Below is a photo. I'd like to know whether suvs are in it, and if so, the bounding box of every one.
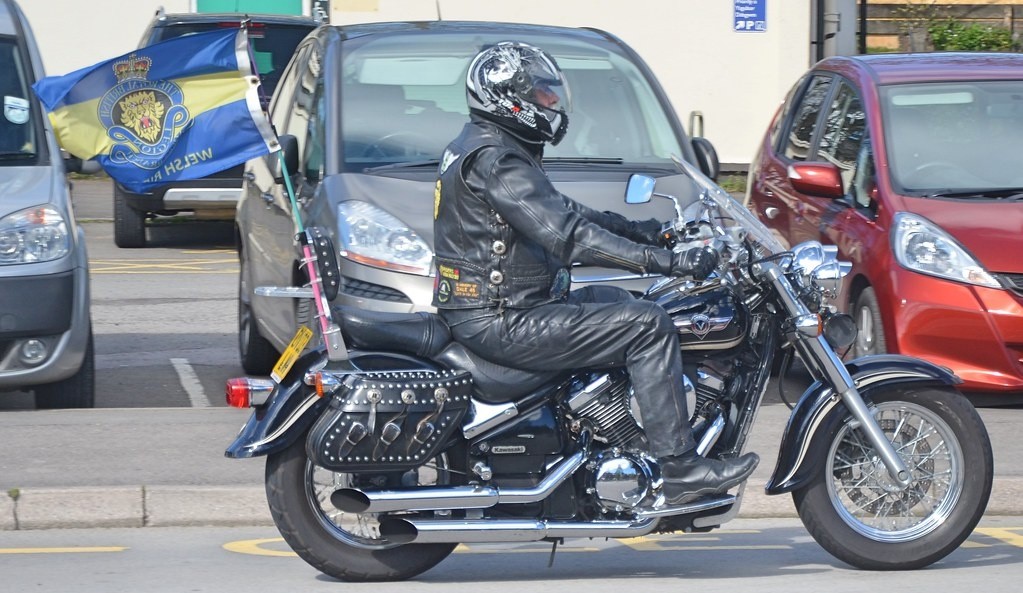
[111,8,330,248]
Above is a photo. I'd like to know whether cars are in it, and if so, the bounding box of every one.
[233,20,802,407]
[743,51,1021,413]
[0,1,97,410]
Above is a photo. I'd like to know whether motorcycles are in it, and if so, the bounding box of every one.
[222,132,993,582]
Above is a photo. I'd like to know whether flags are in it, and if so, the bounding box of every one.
[31,28,282,197]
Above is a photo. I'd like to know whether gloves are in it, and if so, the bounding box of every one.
[671,246,720,284]
[627,217,668,250]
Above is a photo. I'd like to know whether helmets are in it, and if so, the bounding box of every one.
[465,40,572,147]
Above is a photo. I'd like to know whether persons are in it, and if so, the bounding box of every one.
[432,40,760,508]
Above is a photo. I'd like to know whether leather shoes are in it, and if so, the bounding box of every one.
[654,449,762,506]
